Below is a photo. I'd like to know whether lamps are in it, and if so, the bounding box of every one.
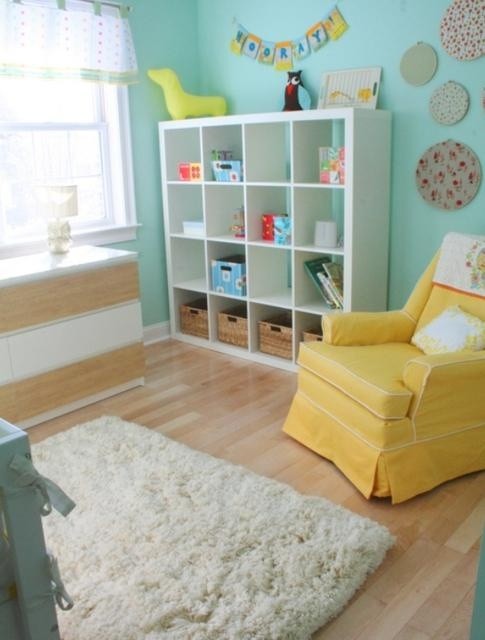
[33,183,80,254]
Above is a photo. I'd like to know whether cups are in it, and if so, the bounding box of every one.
[314,220,337,249]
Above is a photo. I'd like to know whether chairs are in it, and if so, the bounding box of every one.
[282,232,485,503]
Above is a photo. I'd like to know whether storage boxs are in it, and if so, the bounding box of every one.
[210,158,243,182]
[179,298,323,360]
[211,252,246,297]
[317,145,345,184]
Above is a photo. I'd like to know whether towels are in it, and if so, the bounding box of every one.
[432,231,485,298]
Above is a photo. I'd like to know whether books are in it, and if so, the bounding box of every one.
[303,257,343,311]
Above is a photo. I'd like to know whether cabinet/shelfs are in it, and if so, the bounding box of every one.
[156,106,392,373]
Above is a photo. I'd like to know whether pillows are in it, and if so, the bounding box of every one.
[409,303,485,353]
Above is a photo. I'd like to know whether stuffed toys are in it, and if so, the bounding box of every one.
[146,68,228,121]
[280,69,313,112]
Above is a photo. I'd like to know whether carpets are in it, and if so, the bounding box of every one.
[23,414,404,640]
